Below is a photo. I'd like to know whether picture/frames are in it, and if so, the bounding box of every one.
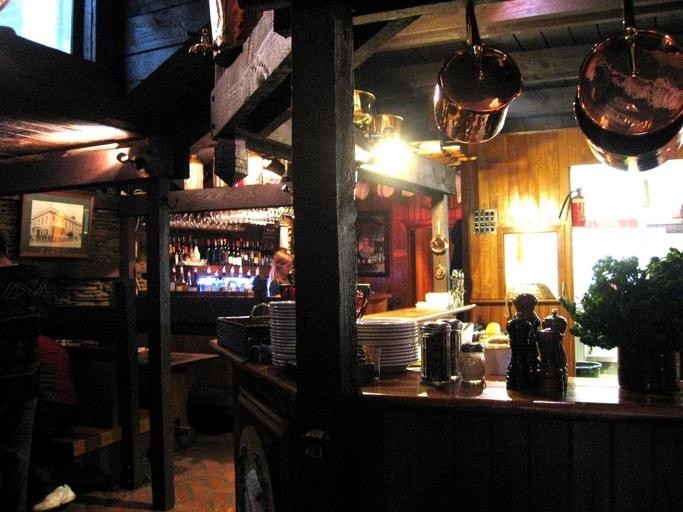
[355,210,389,277]
[18,192,95,260]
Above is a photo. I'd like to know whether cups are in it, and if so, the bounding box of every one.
[169,207,292,231]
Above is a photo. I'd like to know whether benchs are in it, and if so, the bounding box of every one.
[137,332,232,408]
[65,345,150,483]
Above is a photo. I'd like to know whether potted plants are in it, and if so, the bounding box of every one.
[559,248,683,393]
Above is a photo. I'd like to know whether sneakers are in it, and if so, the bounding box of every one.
[32,483,76,512]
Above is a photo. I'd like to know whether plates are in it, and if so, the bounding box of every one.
[270,300,297,369]
[355,317,419,376]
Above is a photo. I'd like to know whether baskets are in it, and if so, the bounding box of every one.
[216,303,270,358]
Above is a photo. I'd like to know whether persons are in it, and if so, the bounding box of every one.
[33,335,77,510]
[0,233,49,511]
[266,249,295,300]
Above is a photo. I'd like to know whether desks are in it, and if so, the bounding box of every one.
[138,349,220,450]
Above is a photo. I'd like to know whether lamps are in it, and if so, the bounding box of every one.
[354,90,405,167]
[281,184,293,196]
[261,158,285,176]
[117,153,143,169]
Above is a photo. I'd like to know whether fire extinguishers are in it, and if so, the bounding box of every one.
[558,187,586,227]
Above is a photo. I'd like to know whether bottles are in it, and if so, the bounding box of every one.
[170,266,263,294]
[460,342,485,385]
[168,231,275,266]
[417,319,460,386]
